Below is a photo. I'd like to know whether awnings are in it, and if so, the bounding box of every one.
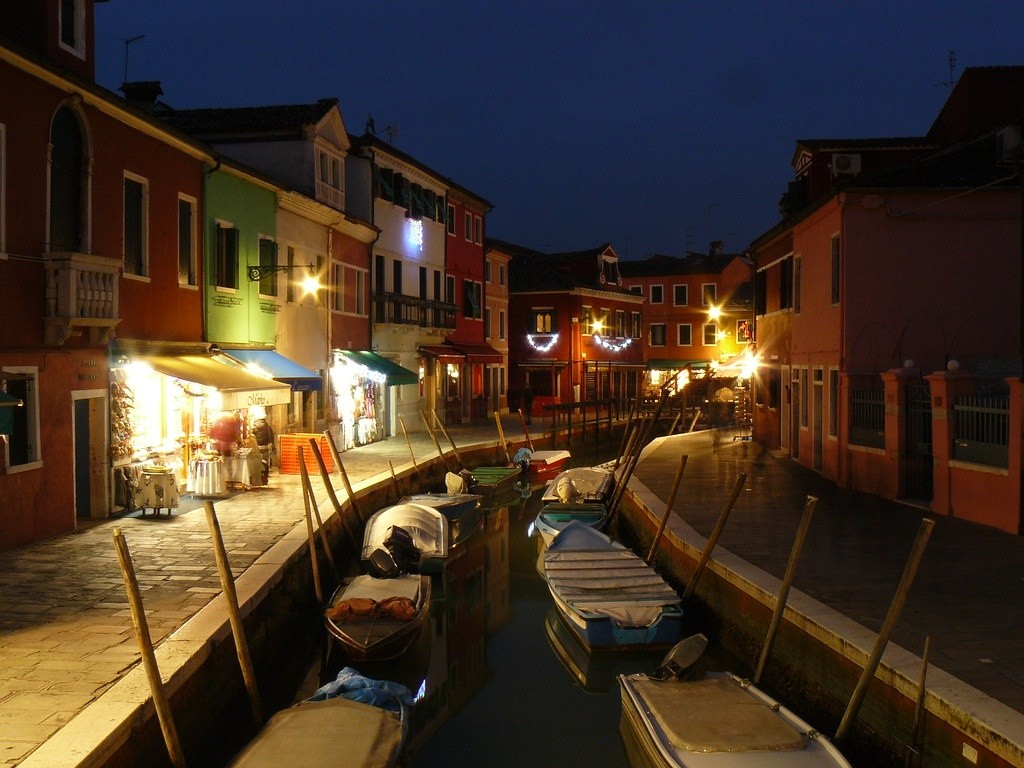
[518,361,568,367]
[136,351,322,410]
[585,361,647,372]
[334,349,419,386]
[645,359,714,370]
[420,345,504,364]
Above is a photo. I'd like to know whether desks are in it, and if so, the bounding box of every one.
[189,459,224,499]
[137,472,176,516]
[220,454,263,492]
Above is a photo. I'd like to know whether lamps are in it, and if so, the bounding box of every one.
[248,261,322,295]
[582,316,603,331]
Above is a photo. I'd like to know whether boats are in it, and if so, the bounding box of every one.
[614,633,852,768]
[534,501,608,547]
[510,449,570,476]
[463,464,523,497]
[322,547,434,666]
[544,518,686,659]
[229,666,412,768]
[541,466,614,507]
[402,493,485,520]
[360,500,449,574]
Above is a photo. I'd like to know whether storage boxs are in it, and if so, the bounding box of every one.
[277,432,335,475]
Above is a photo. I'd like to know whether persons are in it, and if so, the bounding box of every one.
[513,445,532,474]
[210,409,253,490]
[250,417,275,478]
[445,463,468,494]
